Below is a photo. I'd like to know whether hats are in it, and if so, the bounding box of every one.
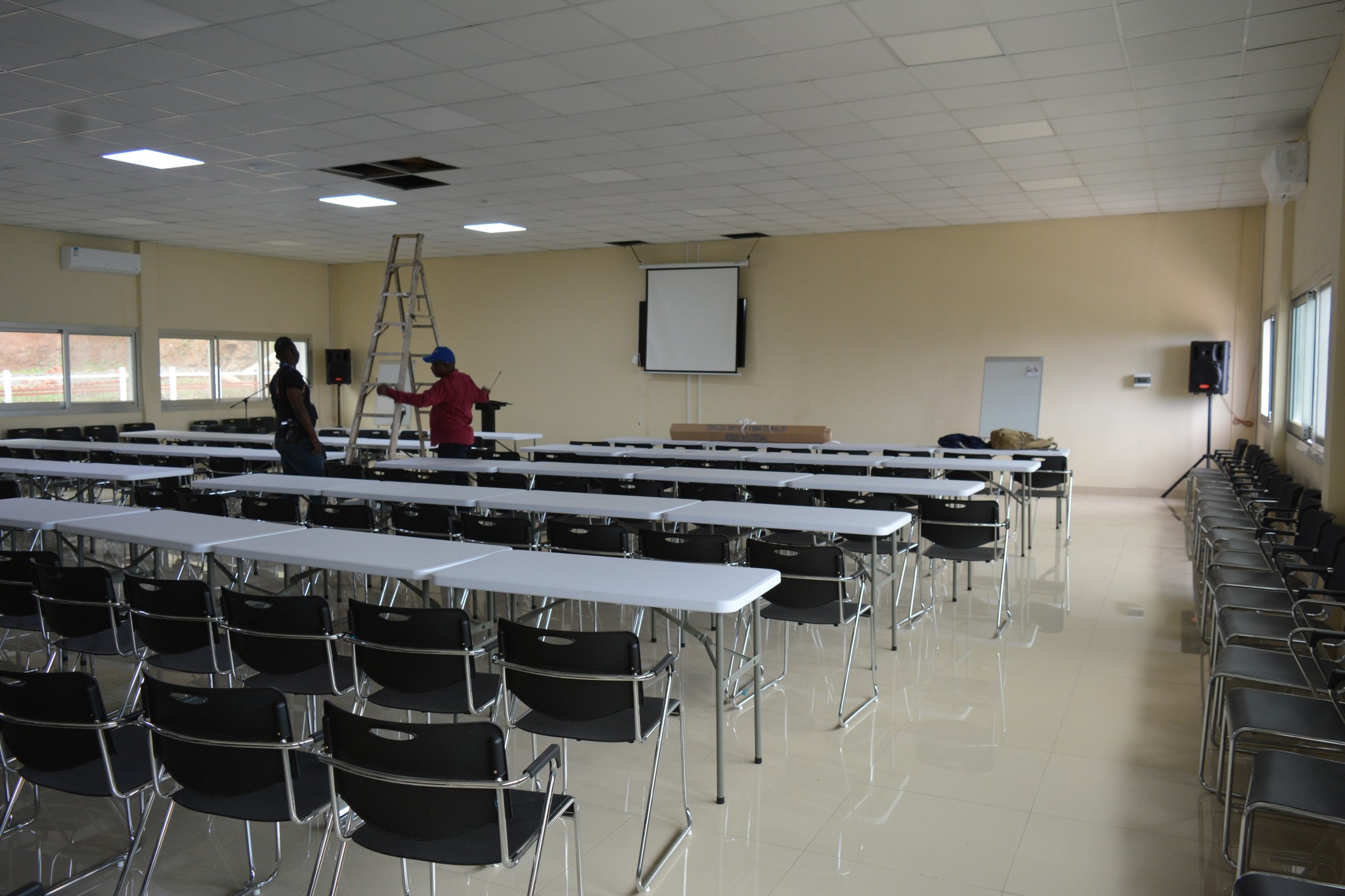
[422,346,456,363]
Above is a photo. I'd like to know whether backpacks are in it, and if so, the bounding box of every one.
[990,428,1058,450]
[937,433,993,449]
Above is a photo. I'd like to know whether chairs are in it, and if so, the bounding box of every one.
[0,414,1075,896]
[1186,437,1345,896]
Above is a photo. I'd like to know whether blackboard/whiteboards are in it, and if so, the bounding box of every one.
[977,356,1044,440]
[372,359,416,426]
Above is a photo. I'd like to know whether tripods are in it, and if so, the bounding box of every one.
[1158,394,1232,499]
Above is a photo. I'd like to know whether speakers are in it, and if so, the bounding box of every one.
[1188,340,1231,396]
[324,348,353,385]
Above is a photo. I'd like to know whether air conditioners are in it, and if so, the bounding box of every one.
[1260,140,1309,200]
[60,244,142,276]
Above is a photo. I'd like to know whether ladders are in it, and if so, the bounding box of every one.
[343,233,443,478]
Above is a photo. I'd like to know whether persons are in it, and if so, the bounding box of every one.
[376,346,491,486]
[268,335,327,526]
